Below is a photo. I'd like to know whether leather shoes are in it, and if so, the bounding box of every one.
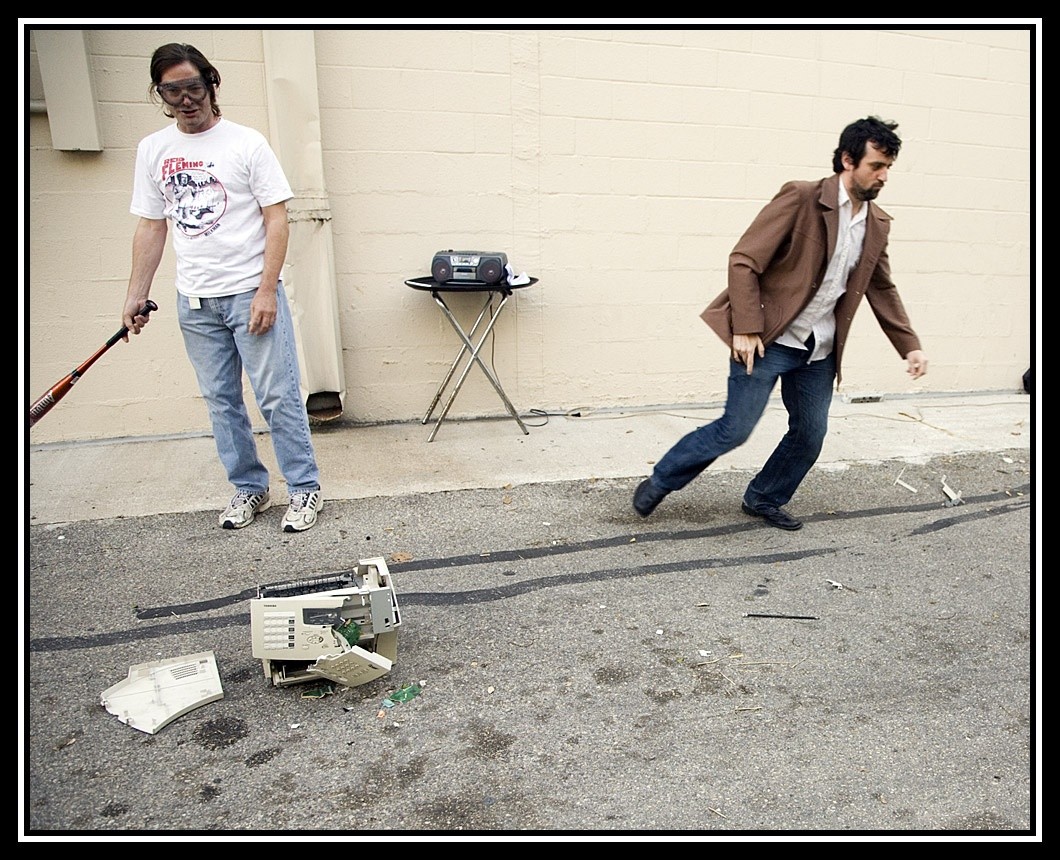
[632,478,665,516]
[741,502,803,530]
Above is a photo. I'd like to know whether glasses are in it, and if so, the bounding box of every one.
[156,79,208,106]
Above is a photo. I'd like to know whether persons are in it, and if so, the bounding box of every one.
[633,116,928,530]
[120,43,323,533]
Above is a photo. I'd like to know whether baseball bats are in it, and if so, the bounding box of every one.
[28,298,159,429]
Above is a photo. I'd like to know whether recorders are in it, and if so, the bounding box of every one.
[431,250,508,285]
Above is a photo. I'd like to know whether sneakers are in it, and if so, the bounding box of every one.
[281,490,323,531]
[218,490,271,529]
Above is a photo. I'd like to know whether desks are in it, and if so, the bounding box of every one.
[405,276,539,443]
